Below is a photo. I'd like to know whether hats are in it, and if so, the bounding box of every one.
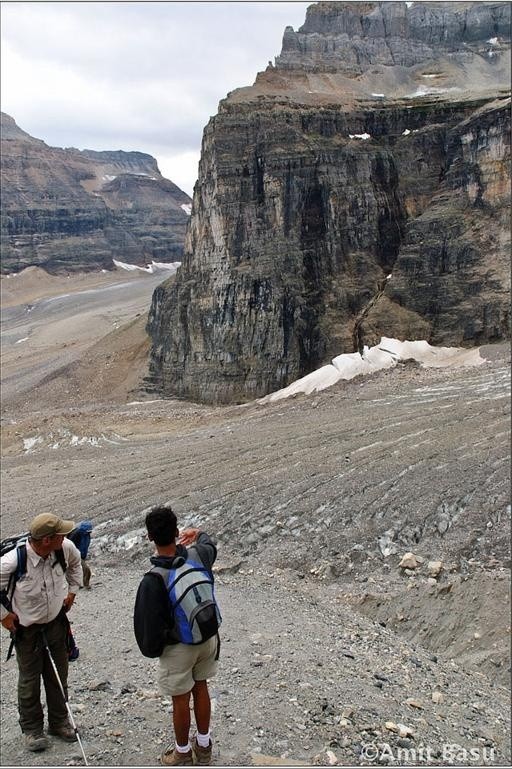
[29,512,75,541]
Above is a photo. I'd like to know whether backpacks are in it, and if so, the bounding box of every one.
[144,546,223,645]
[0,531,67,583]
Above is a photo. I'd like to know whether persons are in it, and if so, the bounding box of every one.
[133,503,222,765]
[1,511,86,752]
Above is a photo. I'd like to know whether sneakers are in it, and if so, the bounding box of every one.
[84,585,91,590]
[47,717,81,742]
[23,730,52,752]
[190,730,213,765]
[160,743,194,765]
